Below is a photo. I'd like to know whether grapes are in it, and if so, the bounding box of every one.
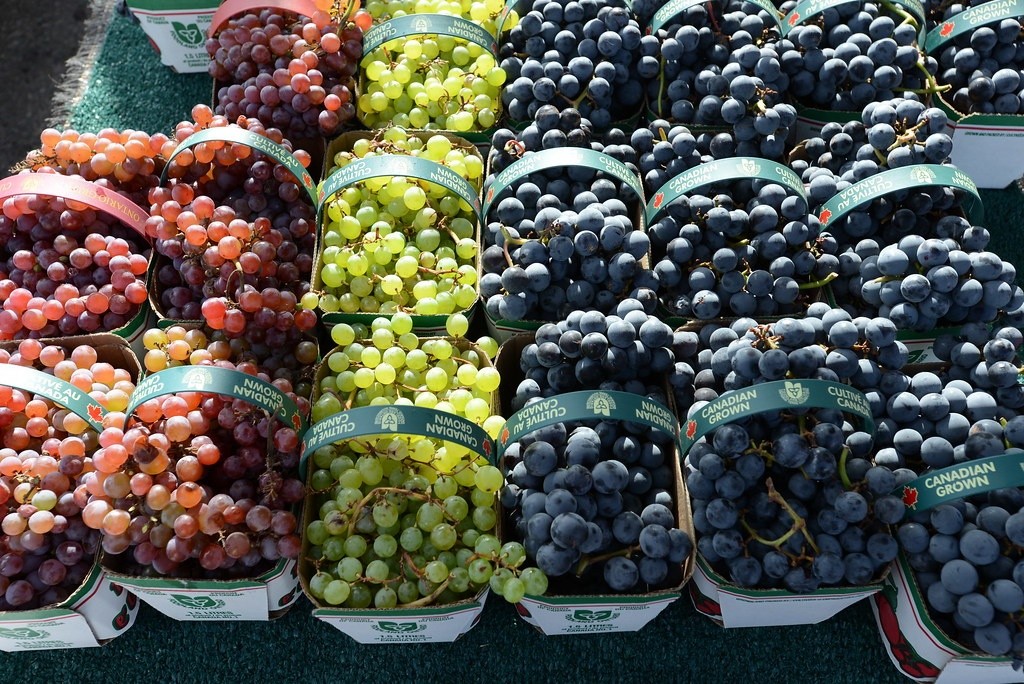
[0,0,1024,657]
